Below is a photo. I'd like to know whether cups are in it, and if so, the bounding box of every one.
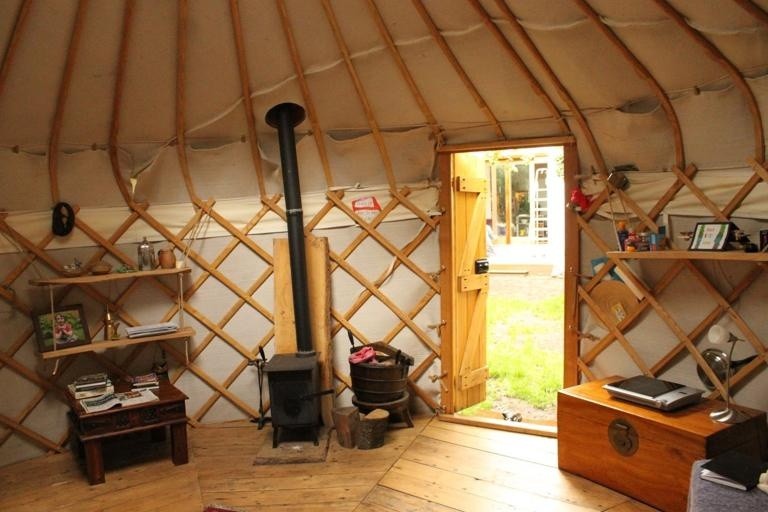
[152,362,168,383]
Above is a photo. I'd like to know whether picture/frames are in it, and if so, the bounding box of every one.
[31,303,91,353]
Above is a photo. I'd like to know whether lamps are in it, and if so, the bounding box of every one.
[704,320,749,423]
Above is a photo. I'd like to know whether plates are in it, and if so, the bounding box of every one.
[696,349,733,391]
[589,276,637,332]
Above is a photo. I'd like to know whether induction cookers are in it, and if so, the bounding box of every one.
[600,373,706,412]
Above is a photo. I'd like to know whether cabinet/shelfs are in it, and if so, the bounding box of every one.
[27,266,196,361]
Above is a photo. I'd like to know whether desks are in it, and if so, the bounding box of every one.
[64,376,190,486]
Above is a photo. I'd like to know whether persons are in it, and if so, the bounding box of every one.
[53,313,80,346]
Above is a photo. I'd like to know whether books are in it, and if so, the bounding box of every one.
[67,370,161,413]
[124,322,178,339]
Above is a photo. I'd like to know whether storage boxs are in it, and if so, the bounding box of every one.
[555,374,765,512]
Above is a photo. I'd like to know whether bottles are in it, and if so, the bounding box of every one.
[614,220,665,253]
[159,249,178,269]
[102,303,121,342]
[138,235,155,272]
